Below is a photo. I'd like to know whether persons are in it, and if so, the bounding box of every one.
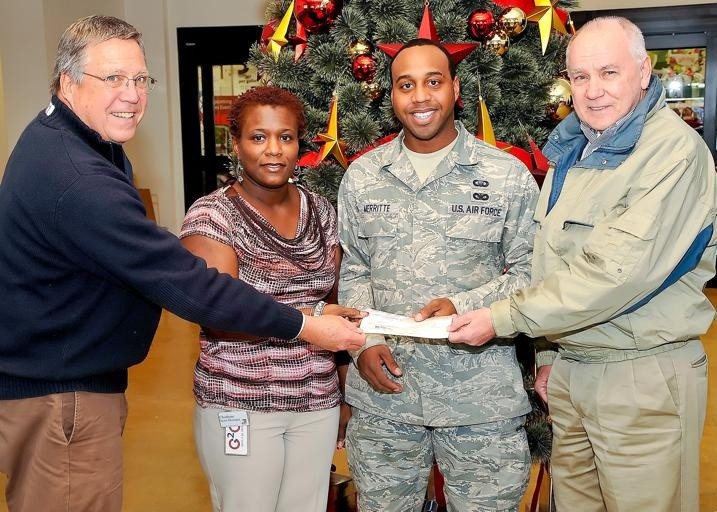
[338,39,541,512]
[681,108,697,121]
[177,86,368,511]
[448,15,716,512]
[1,15,367,512]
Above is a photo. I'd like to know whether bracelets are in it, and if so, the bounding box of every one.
[310,299,327,317]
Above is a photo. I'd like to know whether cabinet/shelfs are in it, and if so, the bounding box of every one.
[664,97,704,134]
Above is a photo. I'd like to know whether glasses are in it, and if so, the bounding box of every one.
[83,72,157,92]
